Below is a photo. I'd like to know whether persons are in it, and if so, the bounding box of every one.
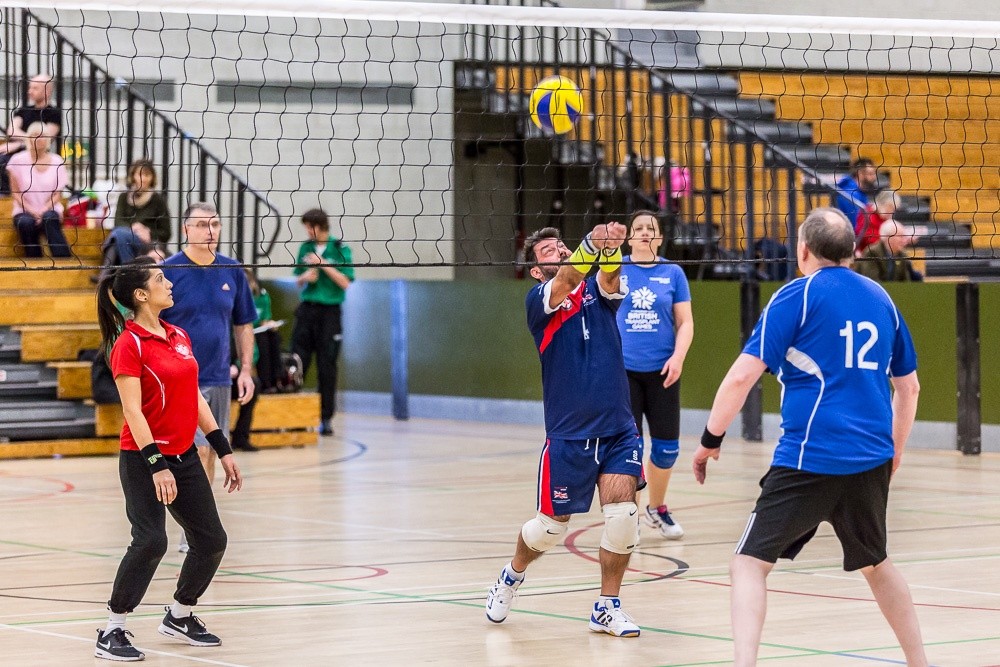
[689,207,926,667]
[290,207,354,436]
[160,202,258,552]
[90,158,171,284]
[93,257,242,661]
[621,154,687,218]
[614,210,695,542]
[0,75,72,258]
[227,266,283,451]
[483,222,645,637]
[831,158,920,283]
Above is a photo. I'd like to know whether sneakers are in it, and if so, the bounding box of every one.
[644,505,685,539]
[95,627,145,661]
[158,604,222,647]
[589,596,640,637]
[486,563,525,624]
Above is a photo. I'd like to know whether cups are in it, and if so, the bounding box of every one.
[85,209,97,231]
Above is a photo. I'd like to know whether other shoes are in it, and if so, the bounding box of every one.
[320,421,334,435]
[231,438,260,452]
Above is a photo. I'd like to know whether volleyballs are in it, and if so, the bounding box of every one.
[529,75,584,135]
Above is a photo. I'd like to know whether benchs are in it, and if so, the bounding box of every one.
[0,195,322,460]
[488,61,1000,283]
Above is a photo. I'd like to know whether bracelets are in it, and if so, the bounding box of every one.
[205,429,233,459]
[139,442,169,475]
[569,231,601,273]
[700,424,726,449]
[599,246,623,273]
